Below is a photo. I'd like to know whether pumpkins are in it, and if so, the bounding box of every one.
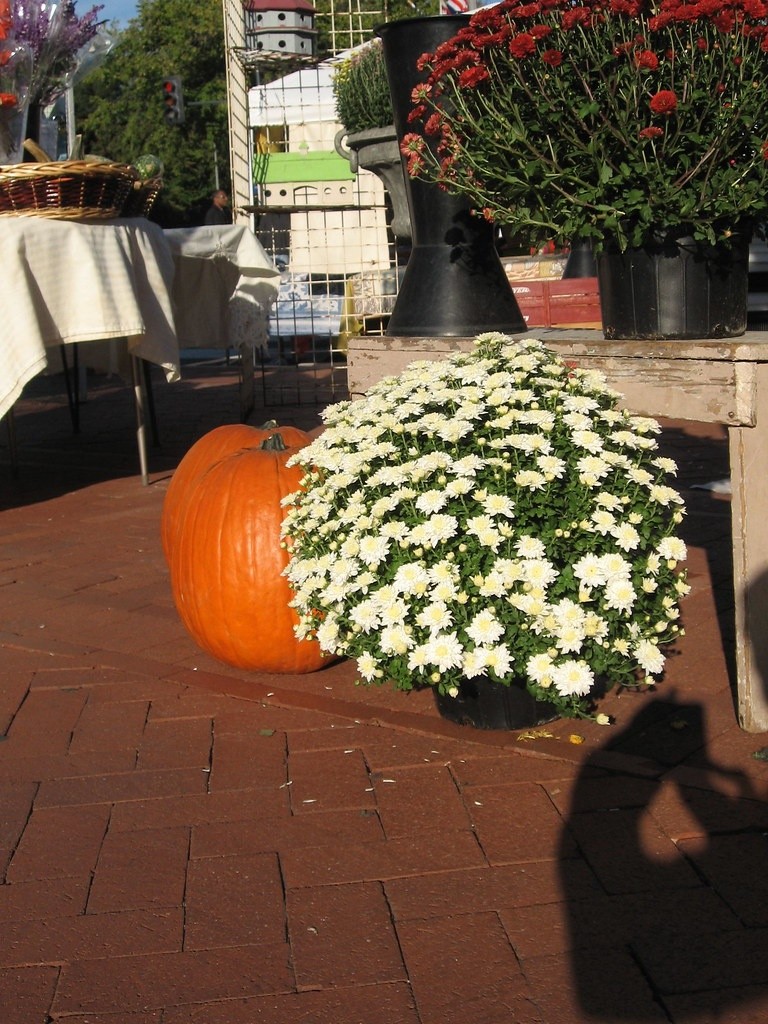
[171,433,347,674]
[161,419,315,572]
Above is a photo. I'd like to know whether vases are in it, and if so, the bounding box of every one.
[592,233,750,341]
[431,668,576,728]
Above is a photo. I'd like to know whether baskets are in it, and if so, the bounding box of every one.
[0,160,163,219]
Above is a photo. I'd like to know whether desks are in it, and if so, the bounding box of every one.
[346,329,768,733]
[59,224,282,452]
[0,218,182,489]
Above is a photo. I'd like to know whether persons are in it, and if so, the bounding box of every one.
[205,190,232,225]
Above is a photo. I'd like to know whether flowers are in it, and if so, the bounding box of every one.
[279,330,691,728]
[398,0,768,257]
[327,39,395,131]
[0,0,109,165]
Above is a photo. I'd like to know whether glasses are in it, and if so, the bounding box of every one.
[214,195,227,198]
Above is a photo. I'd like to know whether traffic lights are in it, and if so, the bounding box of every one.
[161,75,186,125]
